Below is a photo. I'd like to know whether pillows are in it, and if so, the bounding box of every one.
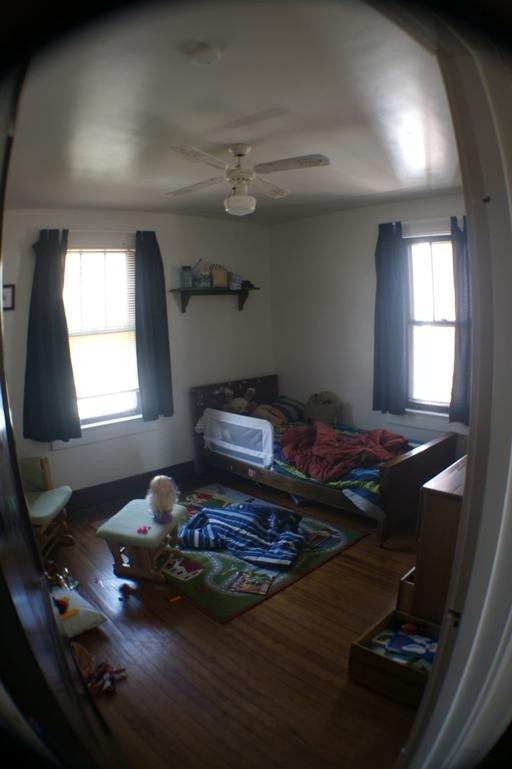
[260,394,306,424]
[49,588,108,639]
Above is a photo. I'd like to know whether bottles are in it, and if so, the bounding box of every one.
[182,264,193,288]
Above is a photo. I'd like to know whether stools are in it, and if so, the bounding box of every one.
[93,499,186,581]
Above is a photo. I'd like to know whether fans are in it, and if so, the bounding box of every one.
[164,142,330,200]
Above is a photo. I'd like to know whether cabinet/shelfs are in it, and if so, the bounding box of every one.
[347,452,470,714]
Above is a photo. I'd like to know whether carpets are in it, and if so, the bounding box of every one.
[124,482,372,627]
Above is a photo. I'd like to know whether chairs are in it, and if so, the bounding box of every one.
[20,456,77,567]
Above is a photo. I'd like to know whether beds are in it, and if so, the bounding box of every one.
[190,373,459,549]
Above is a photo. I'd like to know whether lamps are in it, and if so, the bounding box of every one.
[222,180,257,216]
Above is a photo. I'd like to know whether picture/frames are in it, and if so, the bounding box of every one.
[3,284,15,310]
